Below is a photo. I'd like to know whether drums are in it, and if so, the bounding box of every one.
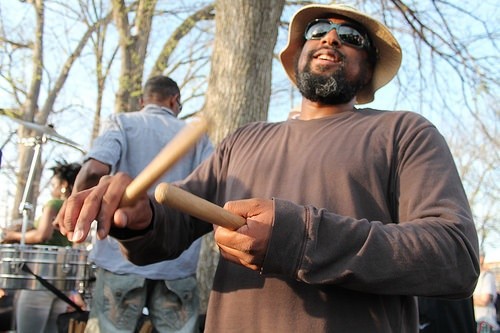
[56,311,156,333]
[477,321,492,333]
[0,243,97,291]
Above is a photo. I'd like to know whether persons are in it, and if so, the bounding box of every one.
[52,76,216,333]
[418,293,477,333]
[472,249,497,333]
[58,4,480,333]
[0,163,82,333]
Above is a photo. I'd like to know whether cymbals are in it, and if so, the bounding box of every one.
[4,114,83,147]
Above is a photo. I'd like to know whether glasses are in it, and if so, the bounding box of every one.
[174,98,182,111]
[305,19,370,49]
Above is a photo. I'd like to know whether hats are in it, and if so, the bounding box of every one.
[280,3,402,104]
